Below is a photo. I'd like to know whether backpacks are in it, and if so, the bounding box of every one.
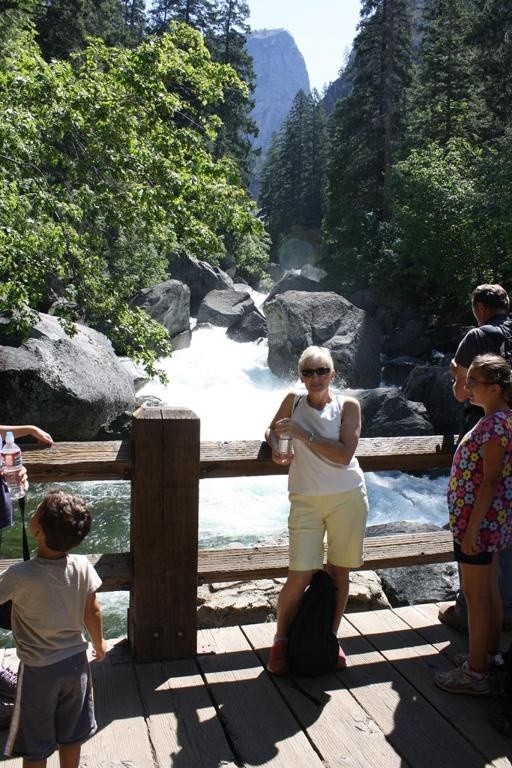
[477,320,512,368]
[283,569,340,687]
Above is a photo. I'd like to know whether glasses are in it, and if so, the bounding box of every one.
[464,378,497,387]
[301,367,330,377]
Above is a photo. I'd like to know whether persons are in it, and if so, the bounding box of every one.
[431,352,512,694]
[0,488,105,767]
[263,344,369,675]
[0,423,54,729]
[439,284,512,637]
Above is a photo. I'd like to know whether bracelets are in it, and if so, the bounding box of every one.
[308,430,316,447]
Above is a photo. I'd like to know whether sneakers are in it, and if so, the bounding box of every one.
[432,601,510,697]
[327,635,349,671]
[267,633,288,673]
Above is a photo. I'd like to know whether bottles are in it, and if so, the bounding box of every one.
[1,431,27,502]
[276,417,292,457]
